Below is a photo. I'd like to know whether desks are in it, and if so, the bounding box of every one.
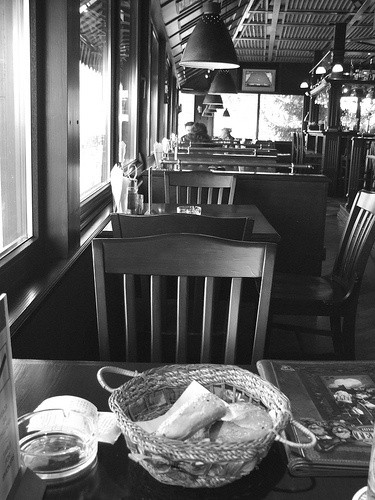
[100,203,282,243]
[7,358,369,500]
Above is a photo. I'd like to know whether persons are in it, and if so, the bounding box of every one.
[219,128,235,148]
[181,122,213,143]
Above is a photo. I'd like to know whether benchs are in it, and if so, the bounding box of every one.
[147,142,328,276]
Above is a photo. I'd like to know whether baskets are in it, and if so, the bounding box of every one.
[97,362,318,489]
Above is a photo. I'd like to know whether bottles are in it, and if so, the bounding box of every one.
[122,134,180,215]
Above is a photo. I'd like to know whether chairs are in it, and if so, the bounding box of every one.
[92,167,375,364]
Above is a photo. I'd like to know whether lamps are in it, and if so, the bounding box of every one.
[178,2,241,117]
[300,80,308,88]
[331,61,343,72]
[316,64,326,74]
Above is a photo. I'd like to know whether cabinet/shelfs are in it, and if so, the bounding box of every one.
[305,131,375,208]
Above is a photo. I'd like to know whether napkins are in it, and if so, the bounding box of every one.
[170,133,178,148]
[152,143,163,167]
[110,163,129,215]
[162,137,170,154]
